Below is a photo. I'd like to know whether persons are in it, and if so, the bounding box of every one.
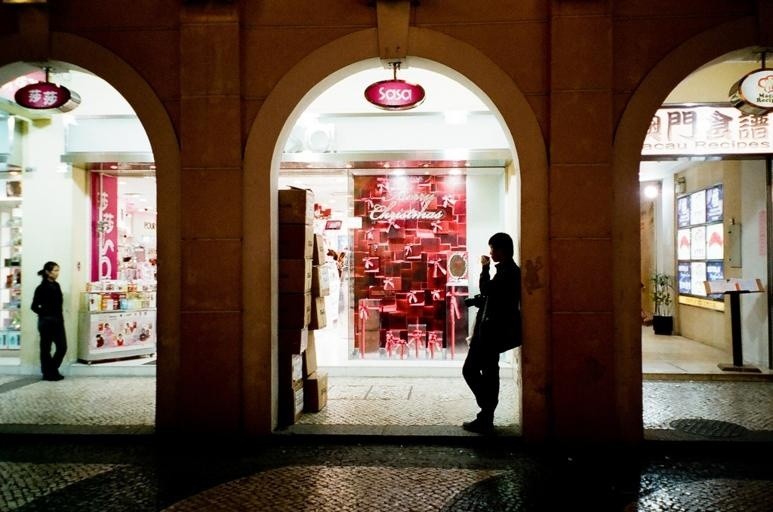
[28,261,67,381]
[458,231,521,434]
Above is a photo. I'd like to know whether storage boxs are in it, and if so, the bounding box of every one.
[277,184,329,428]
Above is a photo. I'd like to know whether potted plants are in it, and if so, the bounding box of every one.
[647,273,677,335]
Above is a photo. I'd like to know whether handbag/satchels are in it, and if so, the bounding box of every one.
[480,311,521,352]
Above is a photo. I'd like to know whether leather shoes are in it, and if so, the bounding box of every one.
[463,422,491,433]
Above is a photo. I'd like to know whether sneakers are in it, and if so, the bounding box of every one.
[42,370,63,380]
[45,359,56,380]
